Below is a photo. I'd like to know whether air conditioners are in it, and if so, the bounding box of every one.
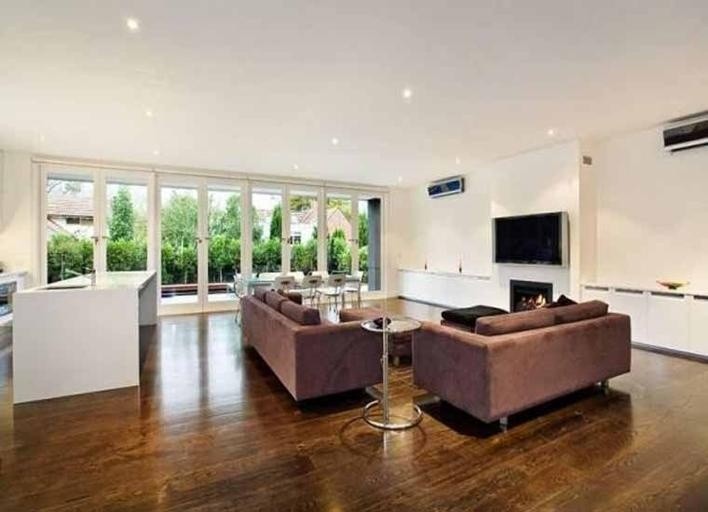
[658,114,708,152]
[423,177,464,197]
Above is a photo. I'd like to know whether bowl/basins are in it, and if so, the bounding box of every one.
[373,317,391,327]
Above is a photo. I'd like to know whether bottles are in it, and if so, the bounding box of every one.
[90,269,96,287]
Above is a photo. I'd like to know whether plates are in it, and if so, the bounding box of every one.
[656,279,689,290]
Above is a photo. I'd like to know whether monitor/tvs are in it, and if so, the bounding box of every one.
[492,211,569,268]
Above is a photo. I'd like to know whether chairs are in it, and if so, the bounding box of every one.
[234,267,366,325]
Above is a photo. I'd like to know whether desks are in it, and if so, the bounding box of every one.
[10,269,161,408]
